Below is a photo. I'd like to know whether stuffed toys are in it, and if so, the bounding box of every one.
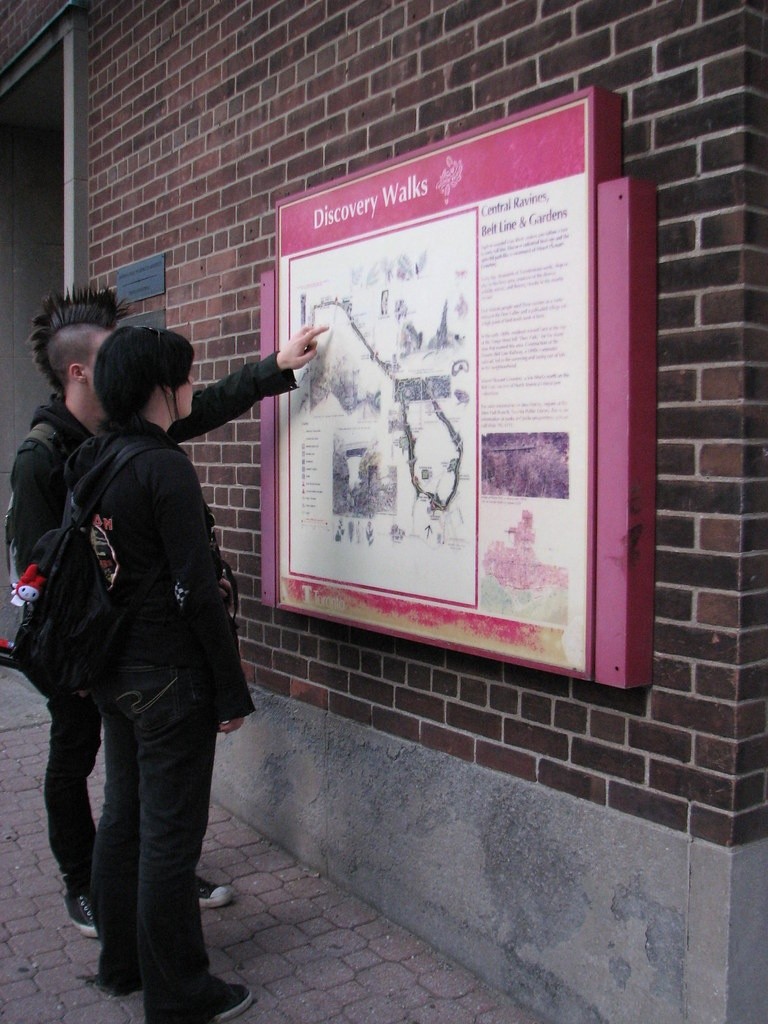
[11,565,46,602]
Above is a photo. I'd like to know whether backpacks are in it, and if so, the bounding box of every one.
[9,437,188,703]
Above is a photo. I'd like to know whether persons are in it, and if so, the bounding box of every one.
[10,290,329,939]
[63,326,256,1024]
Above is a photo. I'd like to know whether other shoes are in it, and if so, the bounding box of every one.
[213,983,251,1023]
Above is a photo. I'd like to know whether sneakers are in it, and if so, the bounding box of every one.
[62,887,99,938]
[196,875,231,908]
